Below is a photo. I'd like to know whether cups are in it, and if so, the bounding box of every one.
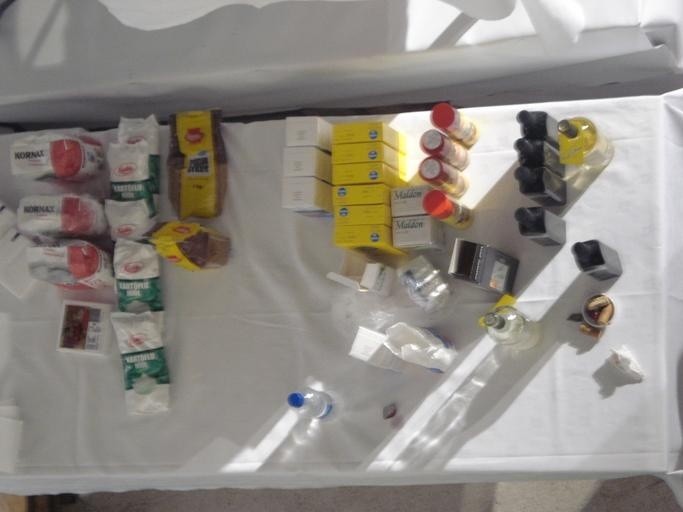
[581,294,617,329]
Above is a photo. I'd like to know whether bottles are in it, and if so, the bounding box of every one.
[288,390,334,420]
[507,110,622,282]
[483,306,539,351]
[418,102,478,230]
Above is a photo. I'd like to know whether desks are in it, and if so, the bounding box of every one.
[1,1,680,124]
[0,87,680,493]
